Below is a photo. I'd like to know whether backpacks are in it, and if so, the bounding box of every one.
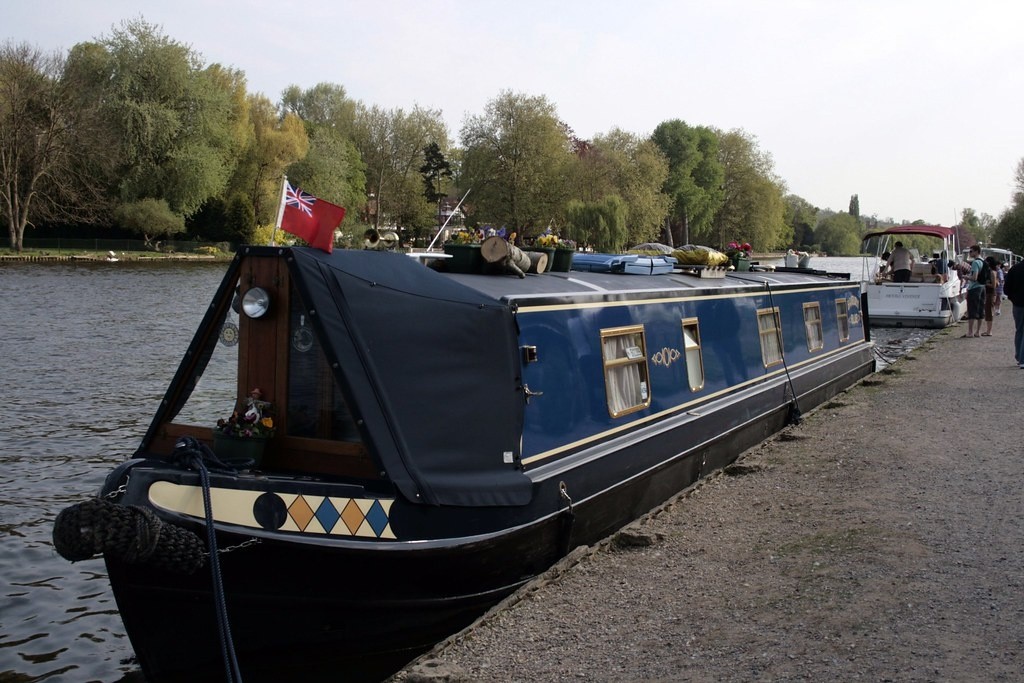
[972,258,992,285]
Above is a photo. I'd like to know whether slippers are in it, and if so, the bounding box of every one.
[981,332,992,336]
[960,334,973,339]
[973,333,979,338]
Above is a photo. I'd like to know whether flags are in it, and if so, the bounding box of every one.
[276,179,346,254]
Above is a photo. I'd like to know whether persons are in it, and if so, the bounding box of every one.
[995,261,1010,315]
[1003,259,1024,369]
[881,252,894,276]
[960,245,986,338]
[947,260,972,299]
[980,256,997,336]
[885,241,916,283]
[931,250,949,281]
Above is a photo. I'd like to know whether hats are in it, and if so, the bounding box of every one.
[947,259,956,267]
[1004,263,1008,266]
[894,241,903,247]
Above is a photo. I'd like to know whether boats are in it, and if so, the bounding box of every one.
[860,224,968,329]
[49,170,877,682]
[962,234,1024,278]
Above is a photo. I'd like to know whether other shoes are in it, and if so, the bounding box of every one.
[996,312,1001,316]
[1016,363,1024,369]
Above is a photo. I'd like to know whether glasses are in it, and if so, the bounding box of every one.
[970,250,975,253]
